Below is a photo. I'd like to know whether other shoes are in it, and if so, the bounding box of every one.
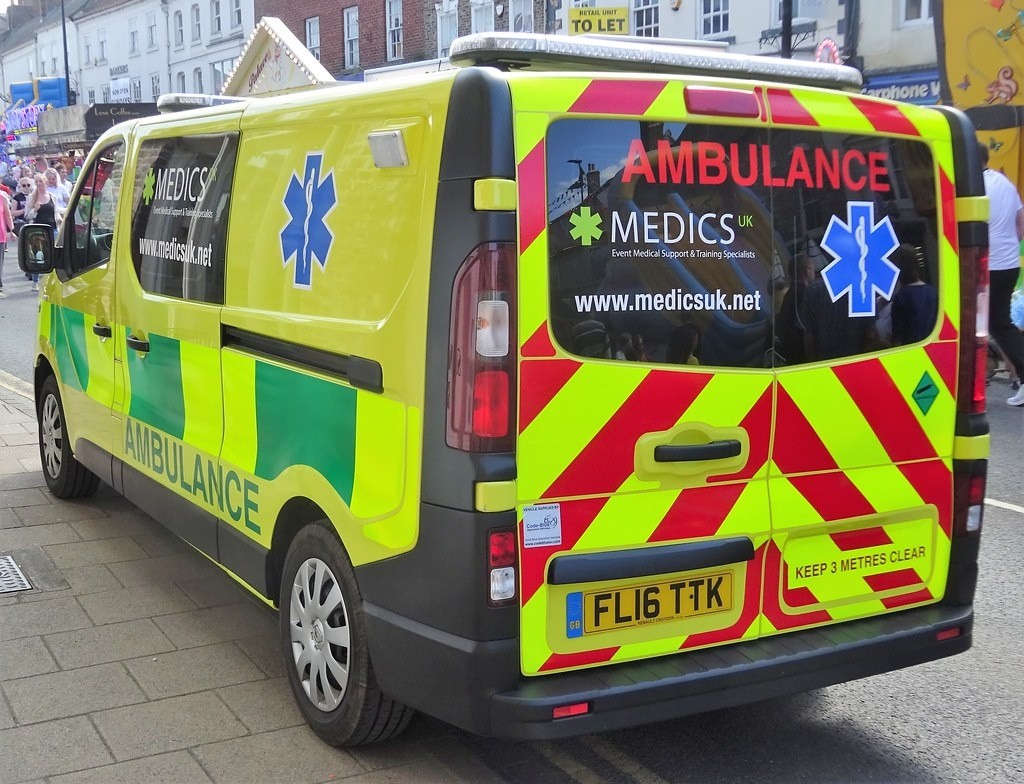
[32,282,38,292]
[0,292,7,299]
[1006,384,1024,405]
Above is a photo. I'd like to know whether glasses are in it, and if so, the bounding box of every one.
[22,184,30,187]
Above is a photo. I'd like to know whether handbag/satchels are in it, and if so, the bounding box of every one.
[12,213,28,237]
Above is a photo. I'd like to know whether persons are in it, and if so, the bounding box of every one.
[23,239,47,266]
[976,140,1024,407]
[0,152,117,244]
[570,304,613,360]
[614,333,636,361]
[675,327,701,365]
[628,336,651,363]
[775,242,938,368]
[23,174,63,292]
[0,191,18,298]
[10,177,35,280]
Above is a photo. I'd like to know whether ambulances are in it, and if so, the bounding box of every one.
[17,30,994,751]
